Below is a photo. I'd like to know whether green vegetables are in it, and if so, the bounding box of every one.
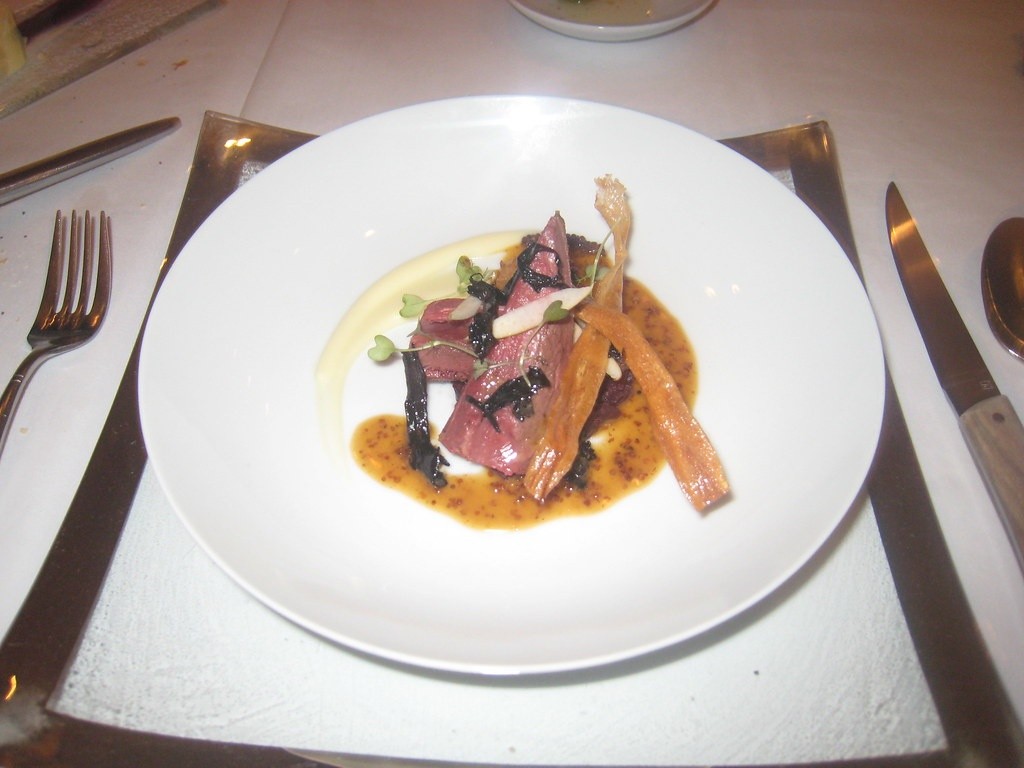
[366,219,621,388]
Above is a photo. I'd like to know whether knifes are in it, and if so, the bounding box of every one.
[0,116,183,206]
[885,180,1024,575]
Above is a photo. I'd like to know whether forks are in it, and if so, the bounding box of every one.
[0,209,114,456]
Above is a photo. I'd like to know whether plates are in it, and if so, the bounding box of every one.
[509,0,714,42]
[136,95,887,677]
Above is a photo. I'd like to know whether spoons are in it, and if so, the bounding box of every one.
[980,217,1024,360]
